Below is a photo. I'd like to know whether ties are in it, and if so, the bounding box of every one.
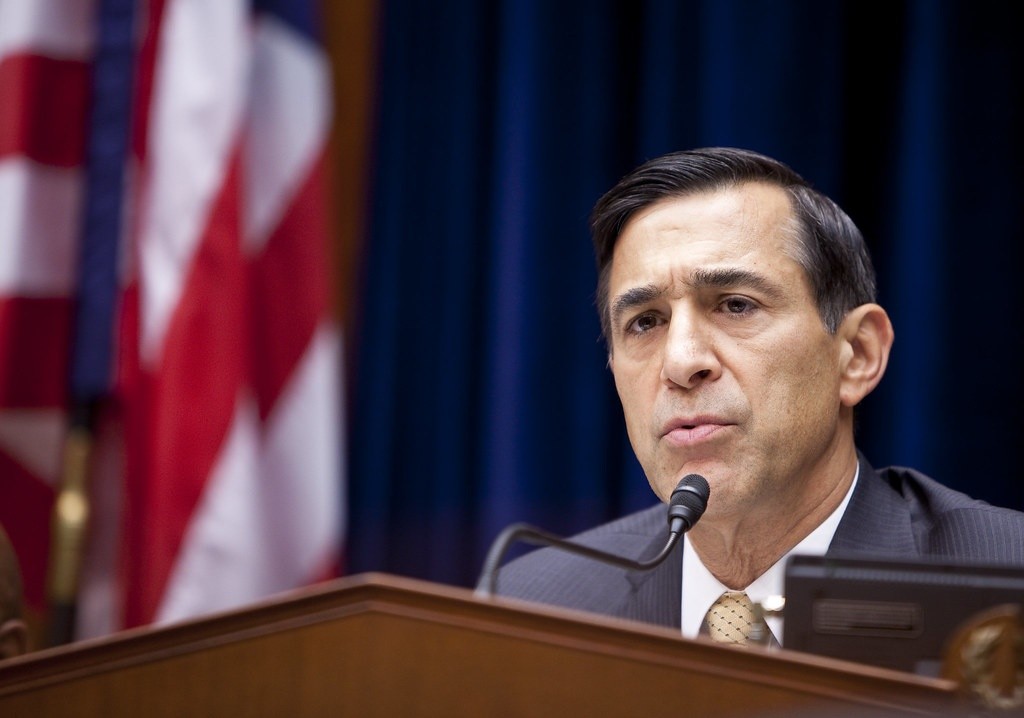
[706,591,767,648]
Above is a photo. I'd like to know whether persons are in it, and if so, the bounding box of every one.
[495,148,1023,650]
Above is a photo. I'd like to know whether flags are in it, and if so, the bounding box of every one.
[0,0,346,637]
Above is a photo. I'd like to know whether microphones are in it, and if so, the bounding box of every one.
[476,475,711,596]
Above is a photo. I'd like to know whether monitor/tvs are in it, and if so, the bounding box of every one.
[784,555,1024,682]
